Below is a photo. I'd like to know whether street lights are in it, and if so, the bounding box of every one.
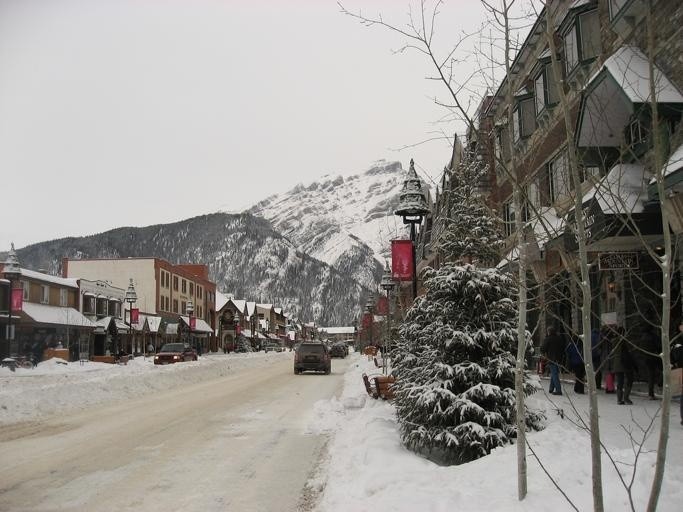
[186,300,194,343]
[0,242,22,372]
[232,311,240,349]
[394,158,432,305]
[125,278,138,359]
[364,290,376,361]
[380,261,396,376]
[358,325,364,355]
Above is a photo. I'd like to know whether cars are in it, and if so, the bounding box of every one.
[265,343,282,353]
[330,344,349,359]
[154,343,198,365]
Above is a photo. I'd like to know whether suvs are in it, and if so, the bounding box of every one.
[294,340,331,375]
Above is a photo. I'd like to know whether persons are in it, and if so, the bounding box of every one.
[537,321,683,425]
[22,337,65,365]
[118,348,128,356]
[146,342,154,353]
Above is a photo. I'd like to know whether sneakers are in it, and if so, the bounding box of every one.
[549,389,562,395]
[617,399,632,405]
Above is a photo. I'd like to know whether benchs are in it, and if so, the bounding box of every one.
[361,372,388,400]
